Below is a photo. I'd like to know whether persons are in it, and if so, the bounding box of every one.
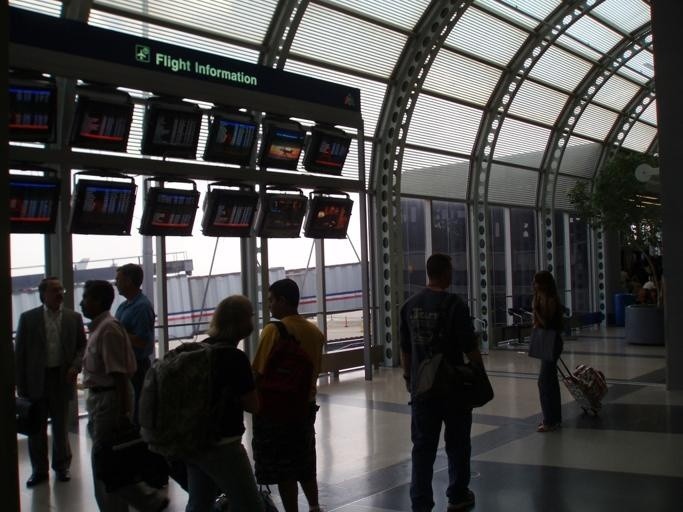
[528,270,563,433]
[79,279,169,512]
[185,295,264,512]
[14,277,88,487]
[251,278,325,512]
[114,264,168,489]
[398,253,484,512]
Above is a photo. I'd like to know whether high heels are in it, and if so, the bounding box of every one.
[538,420,561,432]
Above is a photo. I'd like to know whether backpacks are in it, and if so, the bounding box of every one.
[138,342,237,457]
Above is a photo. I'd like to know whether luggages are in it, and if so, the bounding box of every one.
[556,355,609,414]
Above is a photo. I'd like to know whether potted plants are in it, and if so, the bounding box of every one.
[564,150,663,347]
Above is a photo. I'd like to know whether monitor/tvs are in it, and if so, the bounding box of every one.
[200,181,259,238]
[6,73,57,143]
[258,116,306,171]
[139,175,200,236]
[302,124,353,176]
[70,169,137,235]
[203,104,259,166]
[6,166,62,234]
[140,96,203,160]
[251,185,307,238]
[70,84,134,153]
[302,189,354,239]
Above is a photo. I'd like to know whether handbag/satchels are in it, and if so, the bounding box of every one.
[414,353,494,408]
[215,490,279,512]
[16,397,33,436]
[527,327,560,360]
[254,321,313,428]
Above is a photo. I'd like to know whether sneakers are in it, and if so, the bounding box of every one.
[447,491,475,512]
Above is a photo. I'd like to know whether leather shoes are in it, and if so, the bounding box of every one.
[27,472,48,487]
[56,472,71,481]
